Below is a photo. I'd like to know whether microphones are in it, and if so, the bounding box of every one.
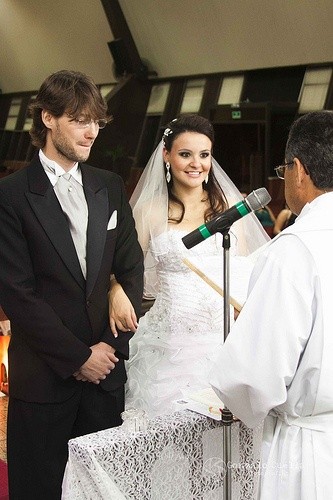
[181,187,271,249]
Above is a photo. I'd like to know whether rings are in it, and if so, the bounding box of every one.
[121,320,125,324]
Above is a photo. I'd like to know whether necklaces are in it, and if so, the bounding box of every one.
[182,201,210,221]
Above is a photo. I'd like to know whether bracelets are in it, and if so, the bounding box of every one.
[110,274,115,280]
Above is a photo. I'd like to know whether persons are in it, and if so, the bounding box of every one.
[0,70,146,500]
[210,112,333,500]
[241,192,297,238]
[108,113,274,422]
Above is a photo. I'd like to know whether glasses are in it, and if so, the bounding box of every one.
[64,110,108,130]
[275,155,311,179]
